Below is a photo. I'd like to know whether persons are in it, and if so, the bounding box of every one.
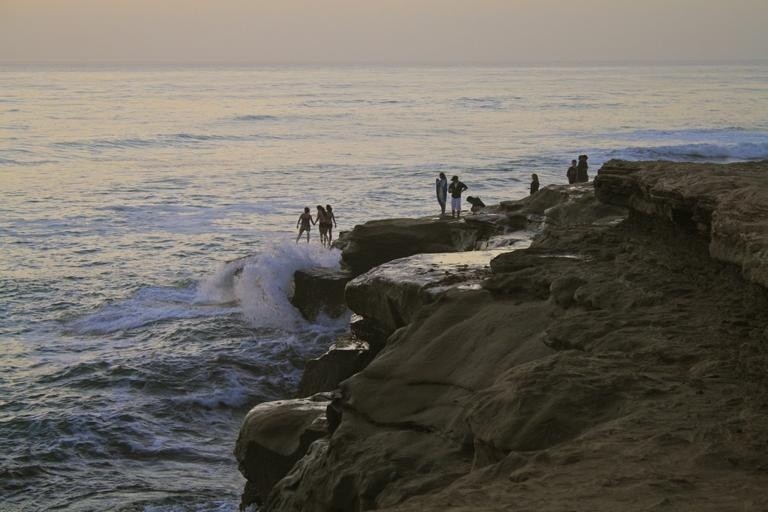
[296,204,336,248]
[567,155,588,184]
[436,173,468,218]
[531,174,539,194]
[467,196,485,215]
